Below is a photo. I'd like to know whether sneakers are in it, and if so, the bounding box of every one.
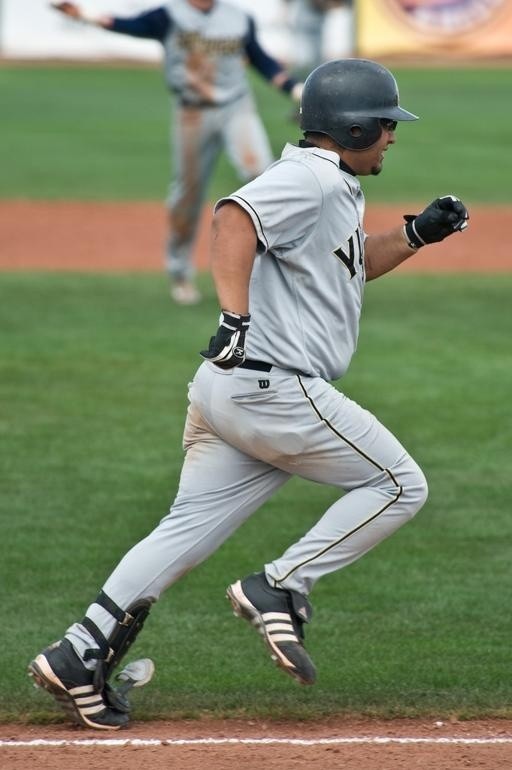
[226,572,317,685]
[27,637,130,732]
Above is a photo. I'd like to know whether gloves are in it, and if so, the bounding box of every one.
[199,309,251,369]
[403,195,469,249]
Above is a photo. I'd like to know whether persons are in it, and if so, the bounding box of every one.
[25,60,470,730]
[49,0,305,303]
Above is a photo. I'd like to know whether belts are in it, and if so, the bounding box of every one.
[237,359,273,372]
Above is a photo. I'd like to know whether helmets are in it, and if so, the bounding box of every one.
[299,58,420,152]
